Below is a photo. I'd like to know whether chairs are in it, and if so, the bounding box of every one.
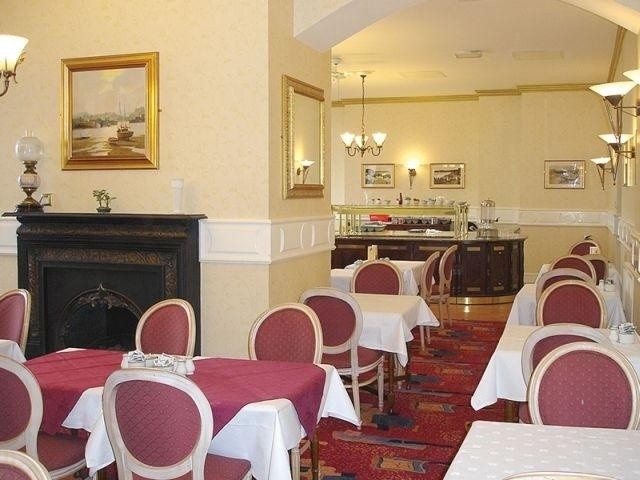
[103,369,253,479]
[0,288,30,355]
[1,355,98,479]
[249,302,324,364]
[135,299,196,356]
[526,342,640,430]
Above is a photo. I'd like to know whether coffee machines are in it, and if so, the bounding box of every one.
[476,198,499,239]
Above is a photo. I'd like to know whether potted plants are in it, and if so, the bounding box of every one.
[93,188,117,213]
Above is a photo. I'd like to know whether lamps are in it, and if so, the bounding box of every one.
[588,69,639,190]
[296,159,316,185]
[14,129,44,212]
[0,35,29,98]
[340,75,387,158]
[407,157,416,190]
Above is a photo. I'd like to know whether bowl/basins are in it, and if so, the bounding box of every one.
[446,199,455,205]
[456,201,467,205]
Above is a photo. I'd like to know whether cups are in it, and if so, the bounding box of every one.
[431,217,438,224]
[122,354,196,376]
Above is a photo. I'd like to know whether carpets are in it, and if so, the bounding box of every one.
[300,319,518,479]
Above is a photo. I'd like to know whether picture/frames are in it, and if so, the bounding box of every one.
[60,52,158,170]
[360,164,395,188]
[429,163,465,189]
[544,160,586,189]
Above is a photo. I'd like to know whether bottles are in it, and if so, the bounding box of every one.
[368,244,379,263]
[172,179,183,213]
[398,192,403,205]
[600,279,605,292]
[453,204,469,238]
[609,323,620,343]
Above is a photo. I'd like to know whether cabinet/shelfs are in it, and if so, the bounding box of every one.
[331,203,528,305]
[1,212,208,361]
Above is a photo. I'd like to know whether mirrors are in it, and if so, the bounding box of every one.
[281,73,326,199]
